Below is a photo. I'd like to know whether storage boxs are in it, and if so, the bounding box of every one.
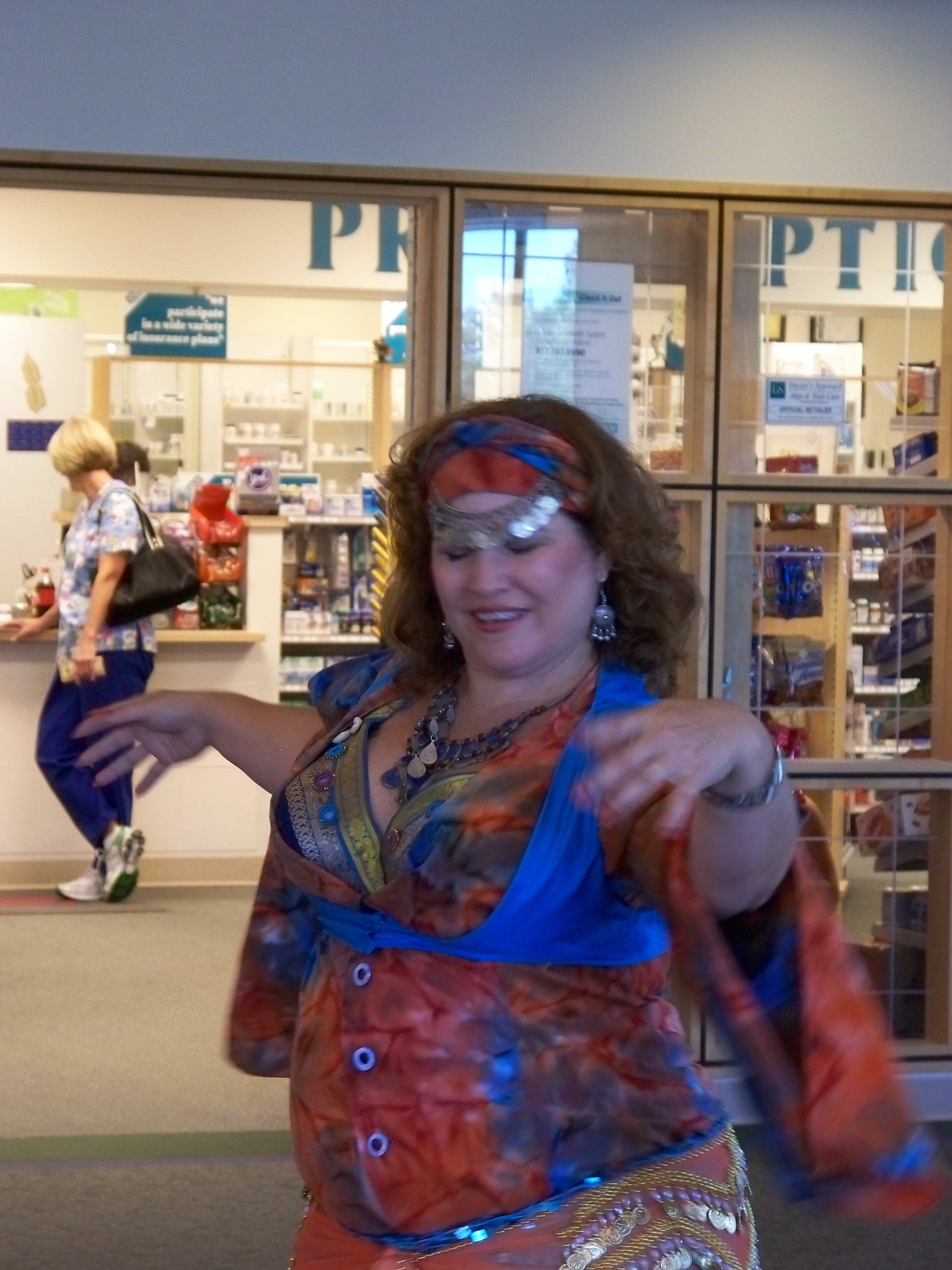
[856,793,932,857]
[882,887,929,932]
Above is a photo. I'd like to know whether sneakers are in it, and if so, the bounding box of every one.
[55,848,106,901]
[103,822,145,902]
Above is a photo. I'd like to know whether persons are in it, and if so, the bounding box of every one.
[71,395,944,1270]
[0,416,156,902]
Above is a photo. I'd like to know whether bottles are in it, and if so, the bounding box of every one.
[852,547,884,581]
[850,598,895,624]
[34,567,55,617]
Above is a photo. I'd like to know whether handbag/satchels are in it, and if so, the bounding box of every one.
[89,488,200,628]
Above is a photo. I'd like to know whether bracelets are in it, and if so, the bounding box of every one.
[701,742,784,813]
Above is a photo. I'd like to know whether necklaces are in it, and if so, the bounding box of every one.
[380,664,574,806]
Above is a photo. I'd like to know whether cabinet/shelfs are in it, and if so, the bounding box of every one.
[870,416,944,953]
[847,524,934,813]
[110,361,407,497]
[278,514,380,692]
[752,463,851,885]
[188,484,248,630]
